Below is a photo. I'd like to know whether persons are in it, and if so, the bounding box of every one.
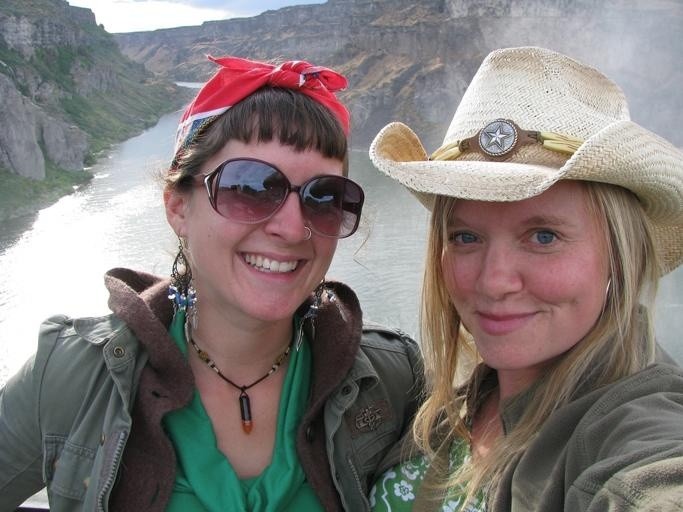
[0,53,426,512]
[366,44,683,511]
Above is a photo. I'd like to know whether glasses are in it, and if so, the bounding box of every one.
[175,154,366,240]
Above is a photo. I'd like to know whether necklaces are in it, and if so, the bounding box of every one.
[183,306,295,434]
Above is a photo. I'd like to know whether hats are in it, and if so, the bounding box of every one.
[367,43,683,286]
